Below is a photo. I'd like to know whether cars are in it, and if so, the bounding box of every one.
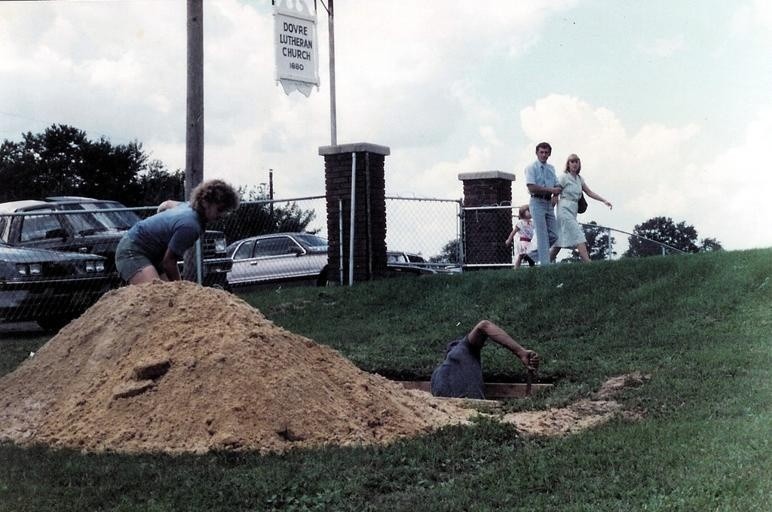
[226,232,328,287]
[0,196,235,330]
[386,251,437,274]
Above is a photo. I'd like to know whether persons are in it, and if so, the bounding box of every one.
[505,205,534,269]
[431,320,539,399]
[523,142,561,267]
[115,179,240,285]
[550,154,613,262]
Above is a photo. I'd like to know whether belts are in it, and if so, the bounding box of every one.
[531,194,552,200]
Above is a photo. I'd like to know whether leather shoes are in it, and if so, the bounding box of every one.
[523,255,535,266]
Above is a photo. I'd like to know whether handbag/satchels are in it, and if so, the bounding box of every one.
[578,195,587,213]
